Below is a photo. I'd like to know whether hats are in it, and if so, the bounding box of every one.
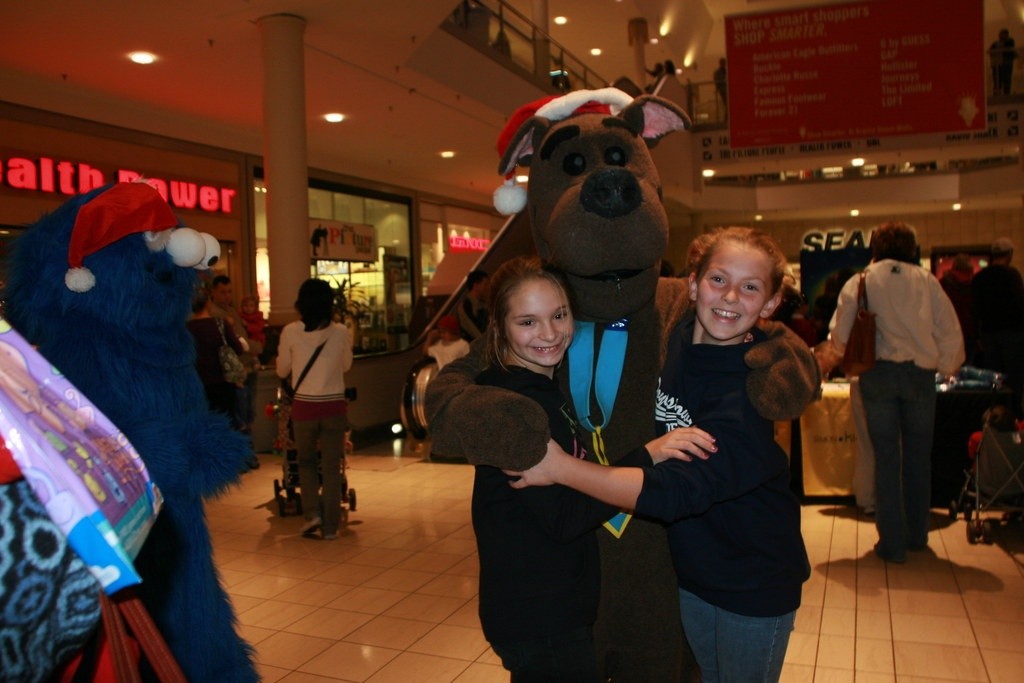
[952,254,974,273]
[992,237,1015,254]
[492,86,635,216]
[839,270,877,376]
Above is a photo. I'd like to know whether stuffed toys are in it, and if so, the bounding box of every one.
[0,181,260,683]
[424,88,820,683]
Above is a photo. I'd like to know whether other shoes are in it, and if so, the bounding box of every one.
[249,457,260,468]
[300,517,322,534]
[908,542,926,553]
[873,540,907,564]
[323,533,336,541]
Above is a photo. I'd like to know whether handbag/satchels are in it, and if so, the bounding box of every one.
[214,316,261,385]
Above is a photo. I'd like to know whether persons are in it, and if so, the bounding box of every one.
[424,228,812,683]
[713,58,729,126]
[644,60,675,94]
[830,222,1024,563]
[185,275,266,474]
[986,29,1016,96]
[275,278,354,539]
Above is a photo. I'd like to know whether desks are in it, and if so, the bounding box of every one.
[773,378,863,501]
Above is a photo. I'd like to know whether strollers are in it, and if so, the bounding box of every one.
[952,374,1024,543]
[272,378,358,517]
